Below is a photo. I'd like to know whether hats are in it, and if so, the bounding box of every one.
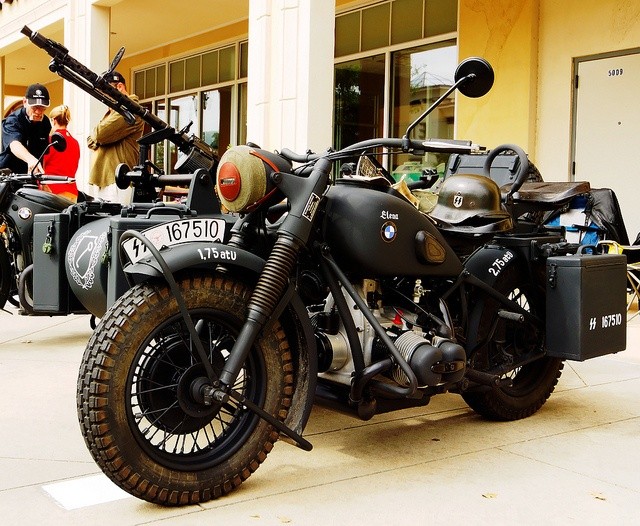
[25,82,50,107]
[101,72,126,83]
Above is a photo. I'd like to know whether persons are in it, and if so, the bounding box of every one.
[0,82,52,175]
[86,71,146,209]
[38,103,81,204]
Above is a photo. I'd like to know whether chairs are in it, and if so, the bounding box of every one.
[596,240,640,325]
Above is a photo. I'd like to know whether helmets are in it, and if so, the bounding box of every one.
[426,174,509,226]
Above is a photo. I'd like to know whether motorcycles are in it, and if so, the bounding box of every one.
[76,57,628,504]
[0,132,75,309]
